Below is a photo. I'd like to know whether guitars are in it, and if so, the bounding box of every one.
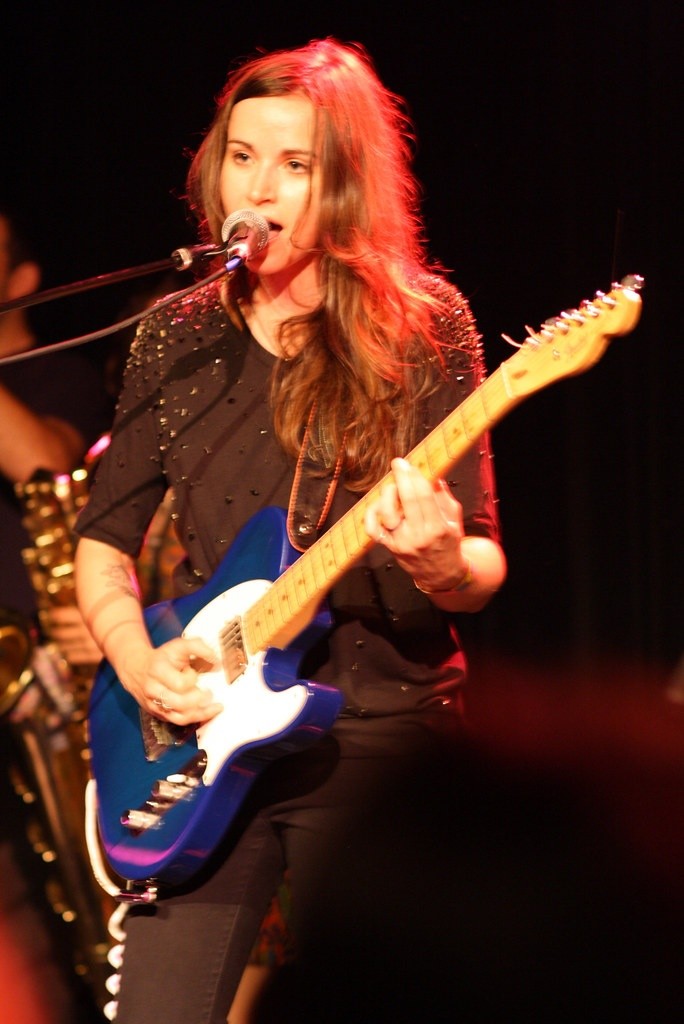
[89,274,645,901]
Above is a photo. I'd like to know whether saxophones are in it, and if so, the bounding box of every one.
[0,467,124,1021]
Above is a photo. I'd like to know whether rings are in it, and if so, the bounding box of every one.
[380,513,403,533]
[153,687,171,710]
[375,530,386,543]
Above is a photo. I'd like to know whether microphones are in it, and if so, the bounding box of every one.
[221,209,270,268]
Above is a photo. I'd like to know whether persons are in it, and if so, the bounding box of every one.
[73,39,504,1024]
[0,213,86,1024]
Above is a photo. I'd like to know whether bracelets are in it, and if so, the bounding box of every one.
[413,555,472,595]
